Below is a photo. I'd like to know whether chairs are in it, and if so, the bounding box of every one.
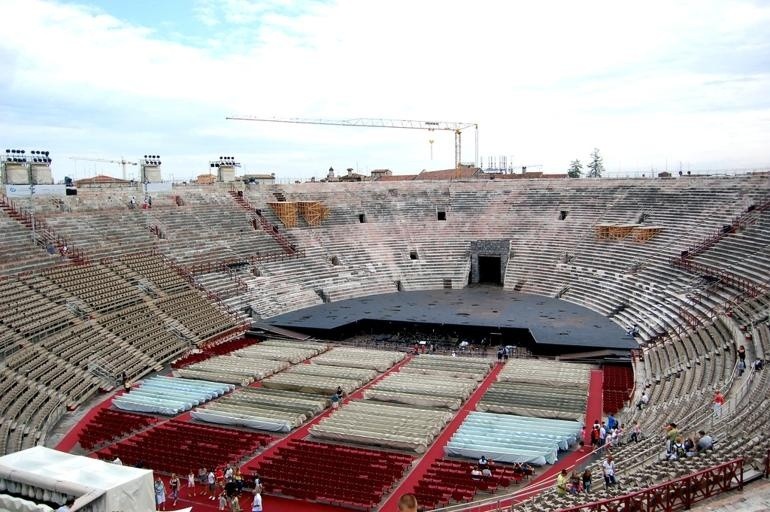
[0,174,770,512]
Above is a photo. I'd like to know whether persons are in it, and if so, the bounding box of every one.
[625,324,639,340]
[129,195,152,208]
[122,369,131,392]
[471,455,534,480]
[47,242,67,256]
[413,337,510,364]
[331,386,343,412]
[713,389,724,418]
[736,345,764,376]
[556,413,713,497]
[636,392,649,410]
[108,453,264,512]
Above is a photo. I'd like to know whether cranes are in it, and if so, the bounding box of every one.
[66,151,139,179]
[221,111,480,178]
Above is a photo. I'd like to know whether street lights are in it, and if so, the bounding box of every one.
[209,156,243,168]
[139,153,163,167]
[4,148,52,164]
